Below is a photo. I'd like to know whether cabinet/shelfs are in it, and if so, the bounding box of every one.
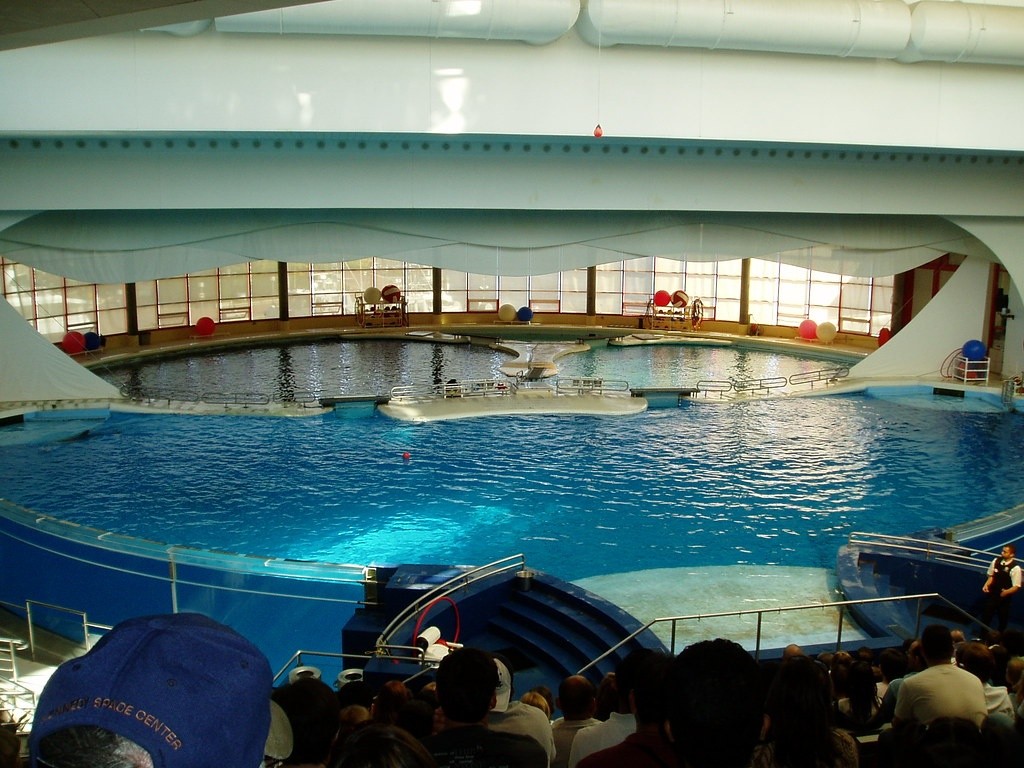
[356,296,409,328]
[651,303,693,332]
[952,356,991,383]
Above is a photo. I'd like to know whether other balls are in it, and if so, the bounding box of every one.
[364,287,382,304]
[84,332,101,350]
[654,290,671,307]
[958,362,978,381]
[381,284,401,303]
[62,331,86,354]
[517,306,533,321]
[498,304,517,322]
[962,339,986,361]
[671,290,689,307]
[194,316,216,337]
[815,321,837,342]
[798,319,818,339]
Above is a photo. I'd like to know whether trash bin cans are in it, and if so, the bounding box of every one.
[140,331,151,346]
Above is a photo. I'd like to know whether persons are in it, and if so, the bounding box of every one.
[976,544,1022,638]
[0,612,1024,768]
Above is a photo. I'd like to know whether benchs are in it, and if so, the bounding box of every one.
[796,337,834,346]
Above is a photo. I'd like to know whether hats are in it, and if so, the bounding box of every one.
[488,657,511,712]
[264,698,294,759]
[31,613,274,768]
[340,704,370,722]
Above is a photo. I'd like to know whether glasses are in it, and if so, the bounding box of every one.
[971,637,983,641]
[988,644,999,650]
[789,655,830,673]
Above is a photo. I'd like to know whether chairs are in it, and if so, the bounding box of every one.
[68,348,104,357]
[189,332,231,340]
[494,320,530,325]
